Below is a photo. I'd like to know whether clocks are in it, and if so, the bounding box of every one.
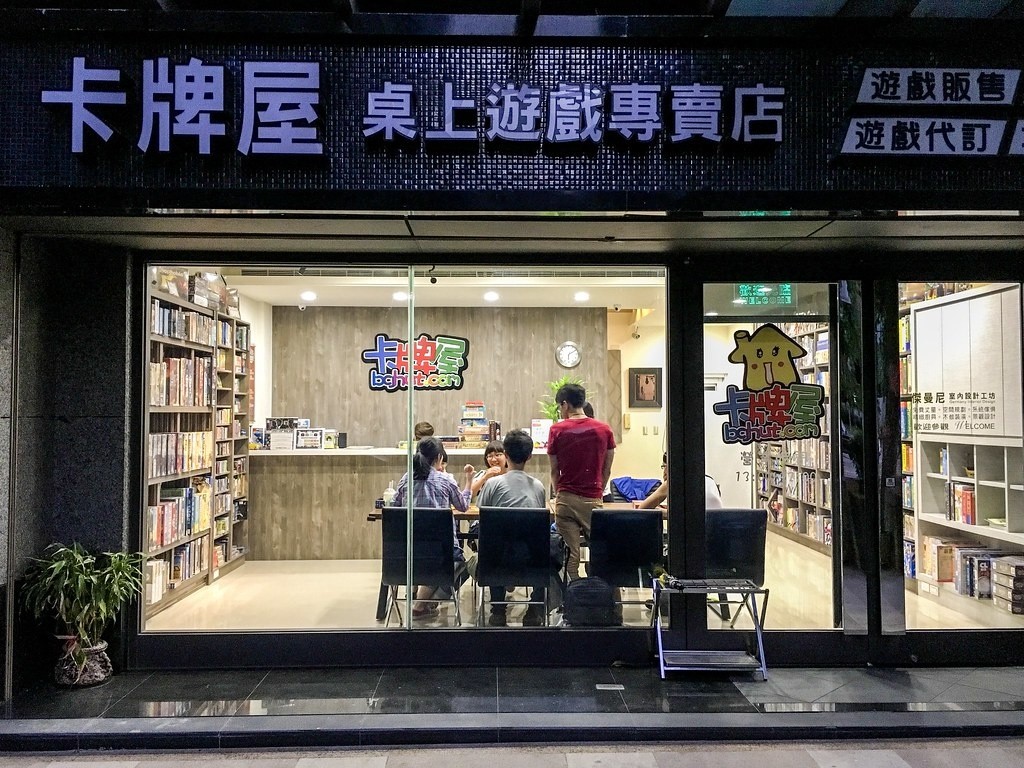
[556,340,582,368]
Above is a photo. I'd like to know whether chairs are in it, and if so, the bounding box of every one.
[476,506,550,628]
[585,510,663,624]
[381,506,463,629]
[705,508,769,619]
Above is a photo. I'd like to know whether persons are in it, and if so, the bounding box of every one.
[406,450,457,600]
[546,382,616,582]
[413,422,434,441]
[389,437,478,621]
[476,428,547,627]
[637,452,723,618]
[466,439,508,523]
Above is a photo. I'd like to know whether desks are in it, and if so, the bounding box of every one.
[365,501,734,620]
[652,577,769,683]
[246,444,555,561]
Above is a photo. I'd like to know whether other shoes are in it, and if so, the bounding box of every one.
[644,598,669,616]
[488,613,543,626]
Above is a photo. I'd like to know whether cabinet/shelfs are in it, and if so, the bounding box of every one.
[899,303,918,583]
[143,287,250,621]
[750,289,832,556]
[912,282,1023,437]
[915,433,1024,546]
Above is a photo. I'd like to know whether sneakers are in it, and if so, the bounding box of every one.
[410,605,438,619]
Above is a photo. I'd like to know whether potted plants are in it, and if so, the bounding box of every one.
[21,539,148,688]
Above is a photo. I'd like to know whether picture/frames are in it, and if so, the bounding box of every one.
[628,366,662,408]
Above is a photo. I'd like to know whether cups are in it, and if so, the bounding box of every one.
[384,487,395,507]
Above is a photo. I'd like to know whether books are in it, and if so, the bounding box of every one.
[756,322,833,548]
[144,265,250,605]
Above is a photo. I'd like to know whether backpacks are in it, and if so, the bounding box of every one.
[563,575,615,628]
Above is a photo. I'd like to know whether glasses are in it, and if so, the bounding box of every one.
[661,464,667,469]
[556,402,563,414]
[486,452,505,461]
